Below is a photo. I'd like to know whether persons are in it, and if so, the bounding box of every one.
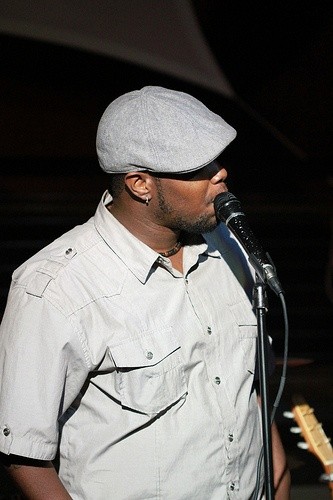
[0,85,291,500]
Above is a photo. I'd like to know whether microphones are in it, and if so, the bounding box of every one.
[214,192,284,297]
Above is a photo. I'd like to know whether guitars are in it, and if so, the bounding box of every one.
[282,404,333,493]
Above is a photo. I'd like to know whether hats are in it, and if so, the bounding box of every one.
[96,84,239,174]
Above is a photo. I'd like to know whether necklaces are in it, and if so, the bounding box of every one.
[159,230,184,257]
